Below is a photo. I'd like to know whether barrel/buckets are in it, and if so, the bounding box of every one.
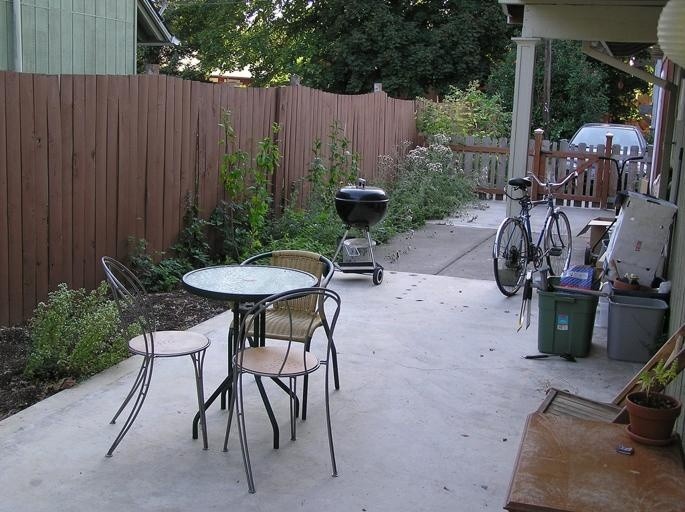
[342,238,376,263]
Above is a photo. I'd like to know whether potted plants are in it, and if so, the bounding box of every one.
[624,358,682,448]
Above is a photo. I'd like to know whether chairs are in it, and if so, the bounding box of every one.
[222,287,340,493]
[102,255,211,457]
[532,323,685,424]
[227,249,339,420]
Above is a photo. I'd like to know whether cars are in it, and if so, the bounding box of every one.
[557,123,654,188]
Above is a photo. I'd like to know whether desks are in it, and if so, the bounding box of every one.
[504,411,685,512]
[181,265,318,449]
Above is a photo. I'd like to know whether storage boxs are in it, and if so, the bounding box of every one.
[576,216,618,254]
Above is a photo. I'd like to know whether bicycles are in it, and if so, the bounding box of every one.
[492,171,582,296]
